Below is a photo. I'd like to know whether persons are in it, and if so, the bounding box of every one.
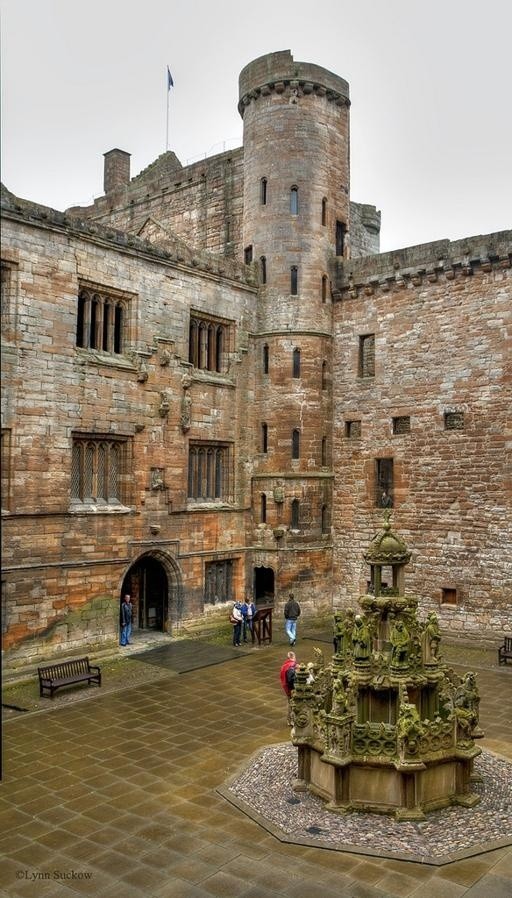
[279,651,297,727]
[331,606,442,667]
[284,592,301,647]
[241,598,257,643]
[232,600,245,646]
[120,594,133,646]
[381,491,393,508]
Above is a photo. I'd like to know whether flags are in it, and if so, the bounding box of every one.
[168,67,174,91]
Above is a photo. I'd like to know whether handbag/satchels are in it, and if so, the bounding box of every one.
[229,614,239,623]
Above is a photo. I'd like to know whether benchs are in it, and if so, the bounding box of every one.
[37,657,102,700]
[499,636,512,665]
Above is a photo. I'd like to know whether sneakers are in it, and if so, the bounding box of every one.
[244,639,257,643]
[287,720,295,727]
[234,643,242,647]
[290,638,296,647]
